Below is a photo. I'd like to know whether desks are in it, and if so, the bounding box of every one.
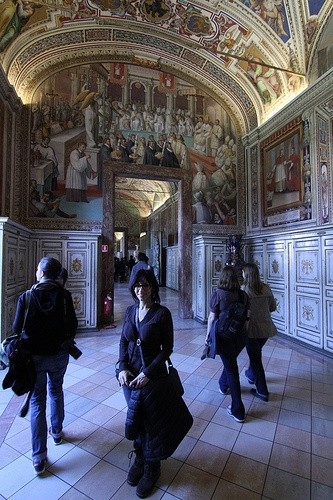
[30,159,60,201]
[48,125,87,182]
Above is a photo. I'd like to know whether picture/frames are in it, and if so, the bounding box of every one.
[262,122,305,216]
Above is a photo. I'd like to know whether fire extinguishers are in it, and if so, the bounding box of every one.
[104,291,112,314]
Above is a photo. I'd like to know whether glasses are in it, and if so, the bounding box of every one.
[133,283,151,290]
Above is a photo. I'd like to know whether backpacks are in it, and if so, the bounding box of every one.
[218,302,247,340]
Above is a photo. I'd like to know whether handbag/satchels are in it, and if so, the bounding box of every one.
[169,367,184,396]
[2,347,35,396]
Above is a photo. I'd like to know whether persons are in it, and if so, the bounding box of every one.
[13,257,78,474]
[127,253,153,290]
[116,269,174,498]
[114,255,135,283]
[240,263,277,402]
[205,266,251,422]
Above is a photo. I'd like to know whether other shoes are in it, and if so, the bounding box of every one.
[226,404,245,423]
[136,469,160,497]
[219,384,229,395]
[53,436,62,445]
[250,388,268,402]
[127,462,144,486]
[34,465,46,474]
[245,370,254,384]
